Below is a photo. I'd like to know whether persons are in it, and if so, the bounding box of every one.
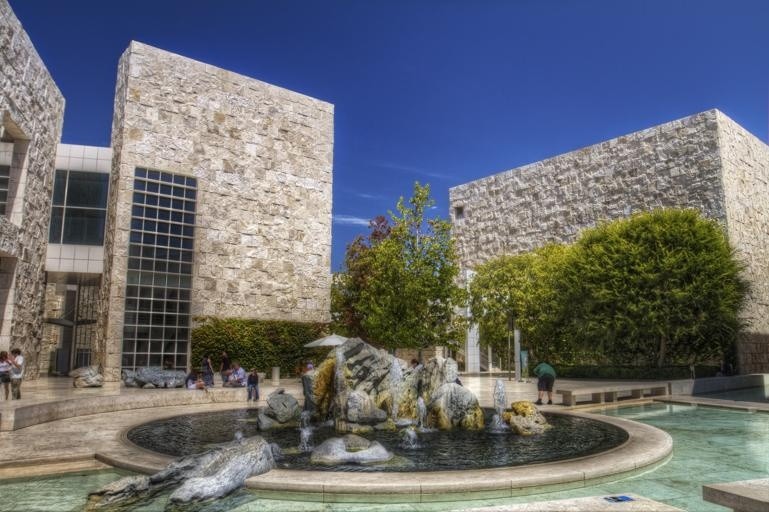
[306,360,315,368]
[7,348,25,399]
[0,351,11,400]
[532,360,556,404]
[186,348,248,388]
[248,368,259,400]
[408,358,422,369]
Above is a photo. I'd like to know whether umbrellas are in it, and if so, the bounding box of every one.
[303,333,355,350]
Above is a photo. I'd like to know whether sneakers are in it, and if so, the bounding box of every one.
[534,398,554,406]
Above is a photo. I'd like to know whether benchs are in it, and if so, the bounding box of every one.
[556,379,667,406]
